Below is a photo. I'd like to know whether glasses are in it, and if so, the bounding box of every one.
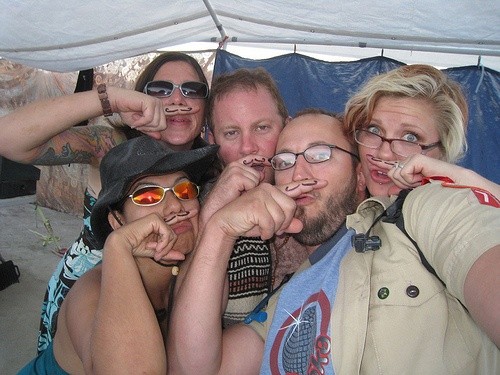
[143,80,209,99]
[268,145,361,171]
[123,181,202,207]
[353,130,441,158]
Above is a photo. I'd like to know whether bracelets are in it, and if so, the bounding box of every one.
[97,84,113,117]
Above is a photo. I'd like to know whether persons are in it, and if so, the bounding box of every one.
[171,68,307,332]
[167,107,500,375]
[340,64,500,200]
[14,134,220,375]
[0,52,211,357]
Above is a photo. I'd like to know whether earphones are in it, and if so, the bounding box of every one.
[246,311,267,325]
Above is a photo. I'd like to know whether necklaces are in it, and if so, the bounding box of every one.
[270,235,292,289]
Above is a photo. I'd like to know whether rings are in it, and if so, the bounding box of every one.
[392,163,403,168]
